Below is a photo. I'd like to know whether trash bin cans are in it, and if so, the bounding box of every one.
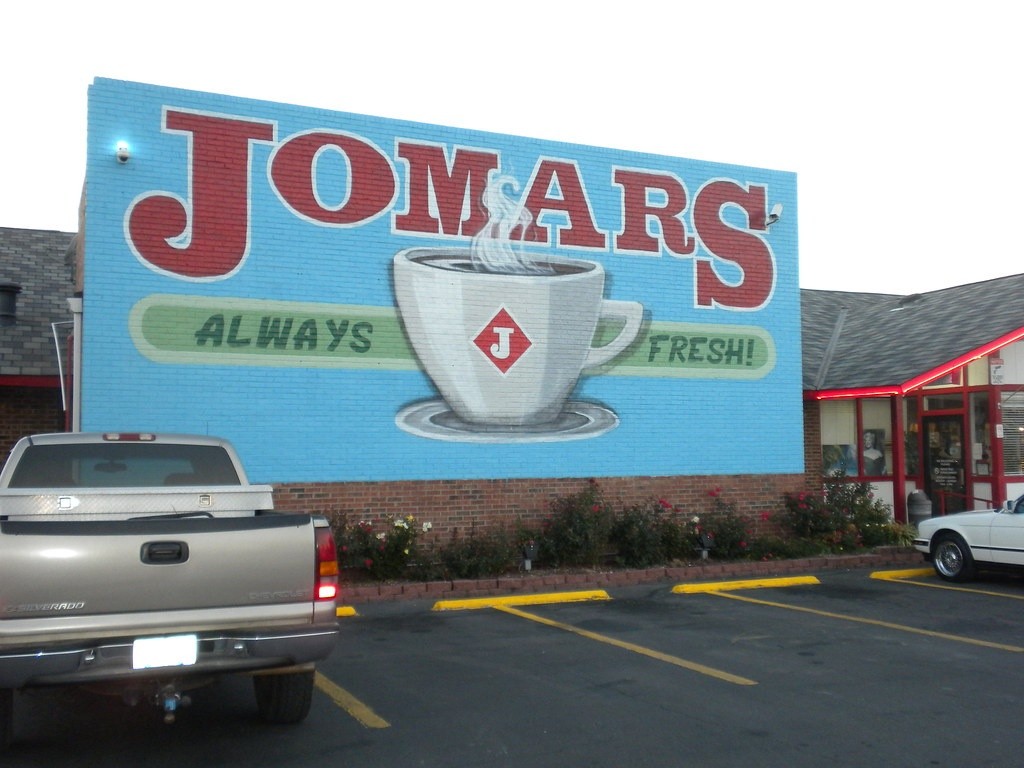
[907,489,932,528]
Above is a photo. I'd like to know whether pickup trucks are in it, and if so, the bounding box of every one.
[0,431,338,734]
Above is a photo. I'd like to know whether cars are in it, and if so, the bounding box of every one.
[910,495,1024,581]
